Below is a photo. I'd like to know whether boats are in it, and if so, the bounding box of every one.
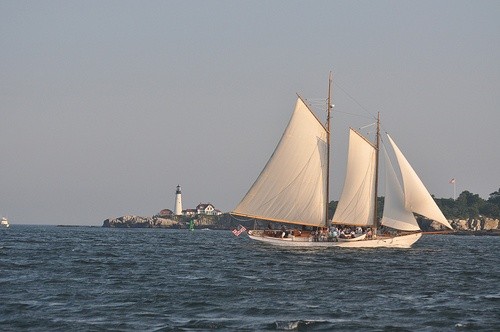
[0,216,10,227]
[200,227,211,230]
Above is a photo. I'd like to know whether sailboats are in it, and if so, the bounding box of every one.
[189,219,196,231]
[226,70,470,249]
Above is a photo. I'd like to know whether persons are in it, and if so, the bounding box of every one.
[281,224,301,239]
[465,214,485,230]
[447,218,462,230]
[302,224,372,242]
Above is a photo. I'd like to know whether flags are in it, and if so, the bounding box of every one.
[449,178,454,184]
[232,223,247,236]
